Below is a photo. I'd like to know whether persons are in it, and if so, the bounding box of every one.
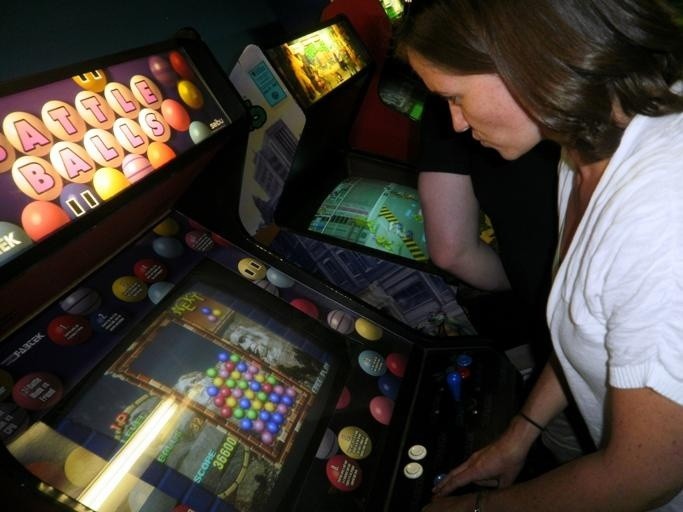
[393,0,683,511]
[412,91,562,296]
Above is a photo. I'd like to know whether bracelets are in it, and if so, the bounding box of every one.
[516,408,546,434]
[471,486,483,512]
